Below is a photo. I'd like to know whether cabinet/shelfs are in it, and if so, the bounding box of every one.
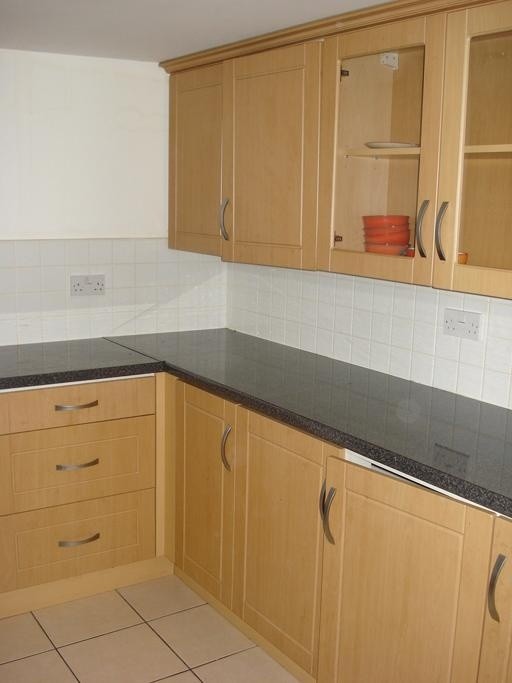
[0,367,165,620]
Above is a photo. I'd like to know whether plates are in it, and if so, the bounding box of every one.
[364,140,418,149]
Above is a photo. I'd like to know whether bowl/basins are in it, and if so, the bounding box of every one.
[361,214,411,258]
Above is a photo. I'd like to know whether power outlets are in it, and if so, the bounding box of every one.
[69,273,107,297]
[443,306,482,341]
